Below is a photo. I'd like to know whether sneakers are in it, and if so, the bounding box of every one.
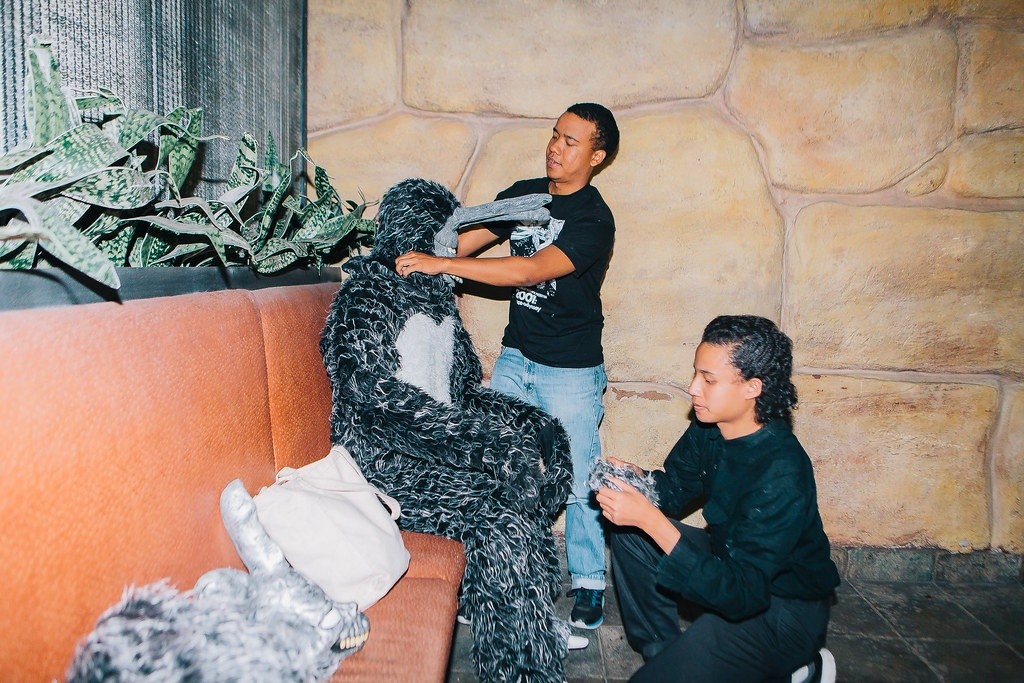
[567,588,605,629]
[457,606,471,624]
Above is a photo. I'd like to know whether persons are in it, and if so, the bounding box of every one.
[395,102,619,629]
[596,315,837,683]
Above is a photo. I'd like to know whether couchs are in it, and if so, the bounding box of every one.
[0,267,467,683]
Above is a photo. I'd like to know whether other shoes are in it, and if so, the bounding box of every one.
[801,647,836,683]
[568,635,589,649]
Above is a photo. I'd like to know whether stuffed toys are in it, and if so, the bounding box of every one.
[319,178,589,683]
[65,478,370,683]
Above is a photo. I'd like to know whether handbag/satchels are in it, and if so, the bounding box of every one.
[251,443,410,614]
